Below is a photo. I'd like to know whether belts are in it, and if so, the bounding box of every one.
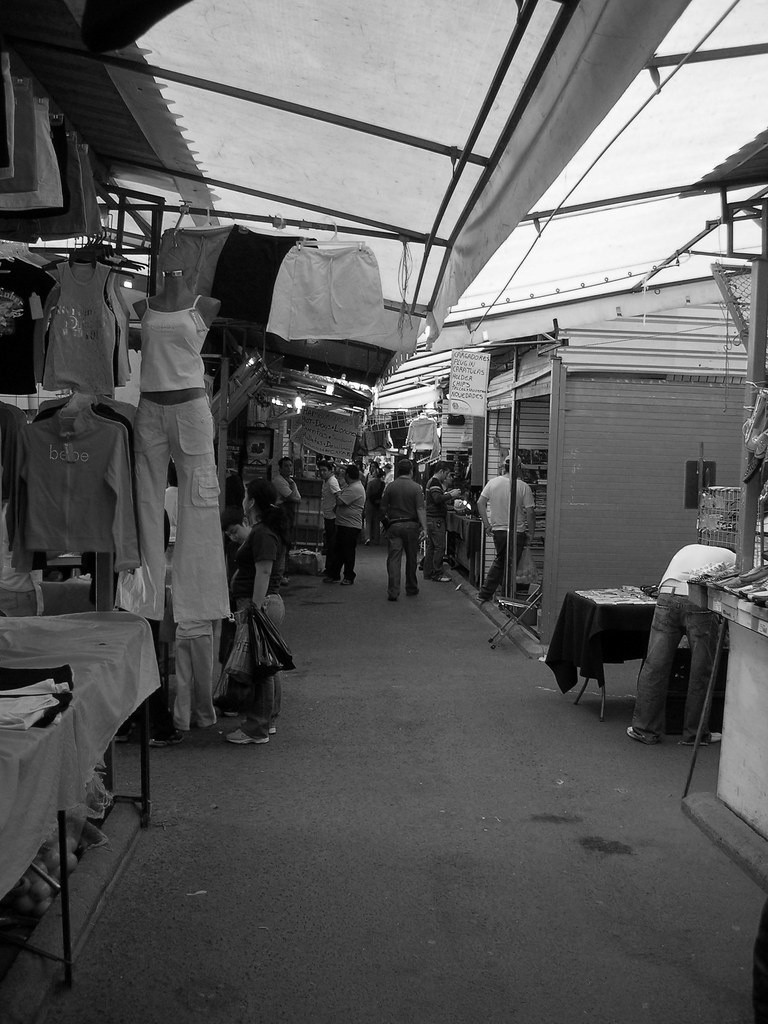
[660,587,688,595]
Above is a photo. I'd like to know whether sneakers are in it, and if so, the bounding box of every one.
[226,720,276,743]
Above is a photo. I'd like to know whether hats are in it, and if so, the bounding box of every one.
[505,454,528,469]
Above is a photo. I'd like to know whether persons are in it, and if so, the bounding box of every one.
[315,452,395,586]
[377,458,429,602]
[626,541,738,746]
[419,461,462,582]
[113,468,287,745]
[476,453,536,602]
[270,456,302,585]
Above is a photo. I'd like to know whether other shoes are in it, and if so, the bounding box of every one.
[711,732,722,742]
[322,576,341,583]
[438,576,451,582]
[365,539,370,546]
[476,595,493,602]
[341,579,354,585]
[686,561,768,607]
[627,727,656,744]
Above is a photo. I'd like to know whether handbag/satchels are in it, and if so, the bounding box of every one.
[213,600,297,713]
[461,421,472,443]
[446,414,465,425]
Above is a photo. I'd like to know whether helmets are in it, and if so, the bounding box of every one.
[454,500,471,515]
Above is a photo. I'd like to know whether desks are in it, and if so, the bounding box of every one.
[0,610,161,986]
[688,582,768,842]
[545,587,660,723]
[445,510,481,585]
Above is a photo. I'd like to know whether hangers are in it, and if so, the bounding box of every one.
[177,208,228,230]
[293,222,366,253]
[243,214,308,240]
[0,226,151,278]
[59,388,100,419]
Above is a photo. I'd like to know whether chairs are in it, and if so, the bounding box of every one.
[487,585,544,649]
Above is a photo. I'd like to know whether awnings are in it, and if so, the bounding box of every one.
[93,0,765,364]
